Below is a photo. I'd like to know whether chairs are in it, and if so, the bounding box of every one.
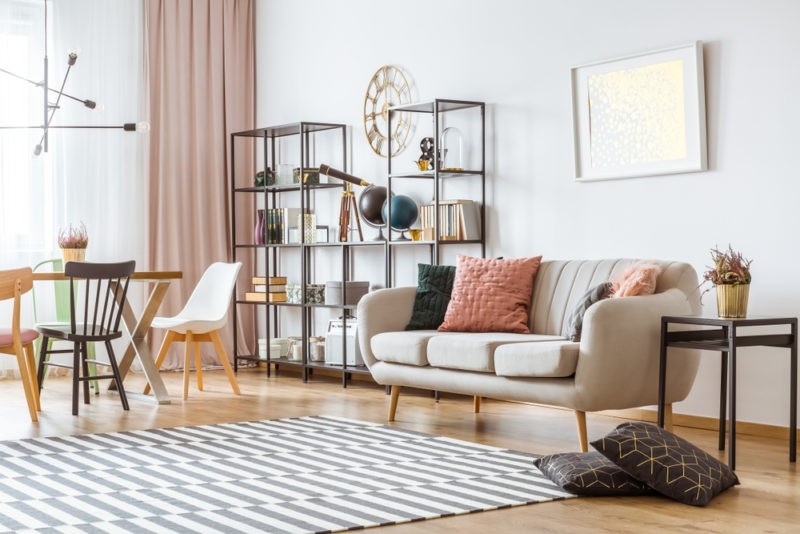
[1,260,242,422]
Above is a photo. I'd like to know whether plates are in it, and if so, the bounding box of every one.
[288,359,302,361]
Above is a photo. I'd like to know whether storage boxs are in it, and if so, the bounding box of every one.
[245,276,287,303]
[293,167,320,185]
[258,339,289,359]
[325,281,369,306]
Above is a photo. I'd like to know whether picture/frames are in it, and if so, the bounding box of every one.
[569,41,707,183]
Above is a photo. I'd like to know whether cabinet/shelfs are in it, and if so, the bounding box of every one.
[229,122,350,383]
[304,239,411,394]
[386,99,487,292]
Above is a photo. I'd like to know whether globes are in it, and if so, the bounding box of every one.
[381,195,419,241]
[358,184,396,240]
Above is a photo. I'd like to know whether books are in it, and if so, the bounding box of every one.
[246,276,288,302]
[419,199,481,240]
[257,208,317,244]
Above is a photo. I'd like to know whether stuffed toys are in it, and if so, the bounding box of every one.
[612,259,661,297]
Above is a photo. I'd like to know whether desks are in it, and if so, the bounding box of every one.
[658,315,798,472]
[31,272,182,405]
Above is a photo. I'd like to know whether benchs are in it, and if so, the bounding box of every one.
[357,258,703,452]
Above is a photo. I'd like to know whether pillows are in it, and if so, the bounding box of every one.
[405,256,541,334]
[566,262,660,342]
[533,422,741,508]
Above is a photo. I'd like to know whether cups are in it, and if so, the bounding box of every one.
[409,229,423,240]
[293,345,303,359]
[313,345,324,361]
[419,161,428,170]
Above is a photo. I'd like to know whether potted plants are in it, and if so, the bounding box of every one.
[684,247,753,319]
[56,220,88,272]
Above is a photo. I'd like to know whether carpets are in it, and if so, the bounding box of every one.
[0,415,580,534]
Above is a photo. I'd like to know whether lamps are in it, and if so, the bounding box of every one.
[0,0,149,161]
